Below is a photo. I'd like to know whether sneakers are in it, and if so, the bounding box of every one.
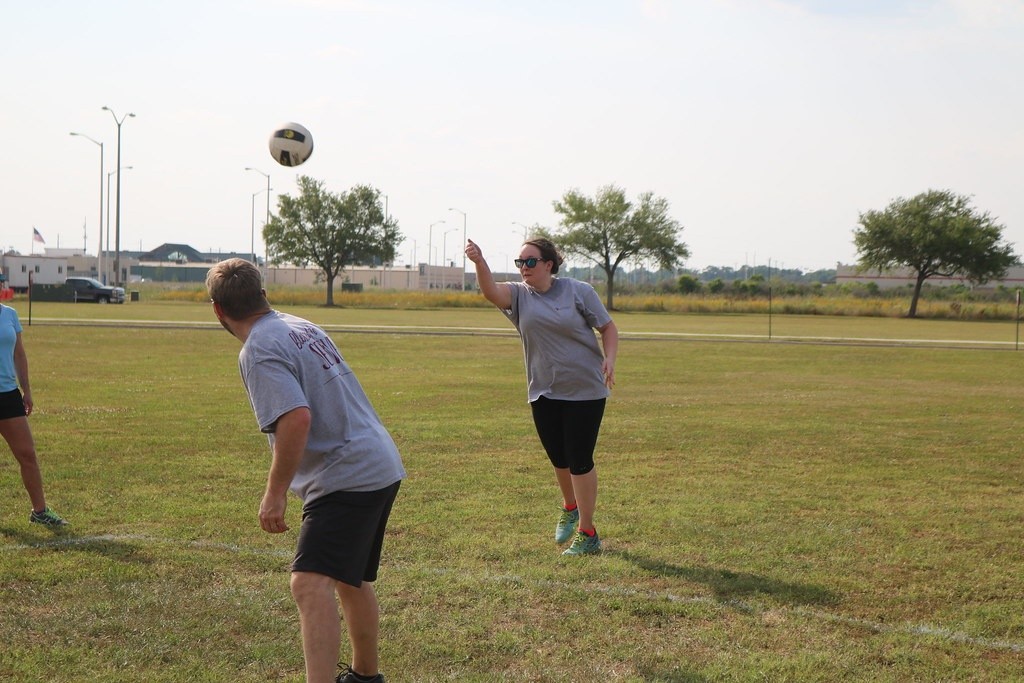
[562,527,601,556]
[28,505,72,528]
[334,662,385,683]
[555,507,579,543]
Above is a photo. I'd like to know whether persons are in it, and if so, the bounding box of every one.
[0,303,70,528]
[465,238,619,556]
[206,258,407,683]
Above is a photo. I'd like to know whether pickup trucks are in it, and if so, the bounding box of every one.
[61,278,125,305]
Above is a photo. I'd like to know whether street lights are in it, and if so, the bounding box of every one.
[513,222,527,240]
[250,189,273,263]
[69,131,103,282]
[447,208,467,292]
[105,166,133,286]
[405,237,416,289]
[427,243,437,289]
[443,228,459,292]
[101,105,136,286]
[498,252,508,282]
[428,220,446,292]
[245,167,270,288]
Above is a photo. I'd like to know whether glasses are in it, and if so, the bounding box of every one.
[514,257,544,269]
[0,274,5,282]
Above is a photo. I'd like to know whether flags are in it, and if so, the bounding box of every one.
[34,229,44,243]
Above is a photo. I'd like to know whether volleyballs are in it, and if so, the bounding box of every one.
[268,121,315,168]
